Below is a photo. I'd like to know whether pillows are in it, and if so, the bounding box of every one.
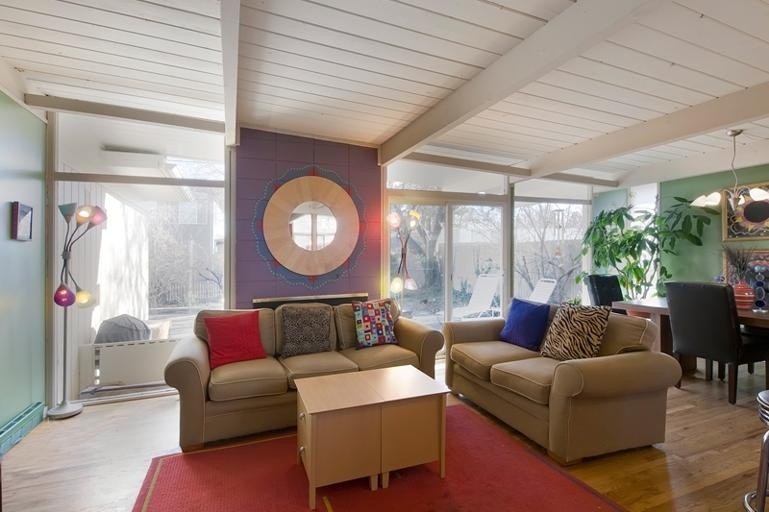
[541,303,611,360]
[201,311,267,369]
[277,304,331,360]
[500,298,550,351]
[353,298,399,351]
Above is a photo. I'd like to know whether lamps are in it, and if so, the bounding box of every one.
[47,203,107,418]
[386,210,419,315]
[690,129,769,210]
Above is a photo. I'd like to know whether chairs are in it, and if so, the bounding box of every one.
[462,278,557,321]
[433,273,501,322]
[662,281,769,405]
[585,275,627,315]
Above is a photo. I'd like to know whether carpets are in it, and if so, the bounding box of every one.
[132,402,626,509]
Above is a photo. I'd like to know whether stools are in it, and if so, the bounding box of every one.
[741,390,769,512]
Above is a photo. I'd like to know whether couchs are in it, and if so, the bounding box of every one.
[443,297,683,464]
[164,304,445,452]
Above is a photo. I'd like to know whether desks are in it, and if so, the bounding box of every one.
[611,297,769,352]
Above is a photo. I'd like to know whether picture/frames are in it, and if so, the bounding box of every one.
[13,202,33,241]
[722,181,769,240]
[723,248,769,312]
